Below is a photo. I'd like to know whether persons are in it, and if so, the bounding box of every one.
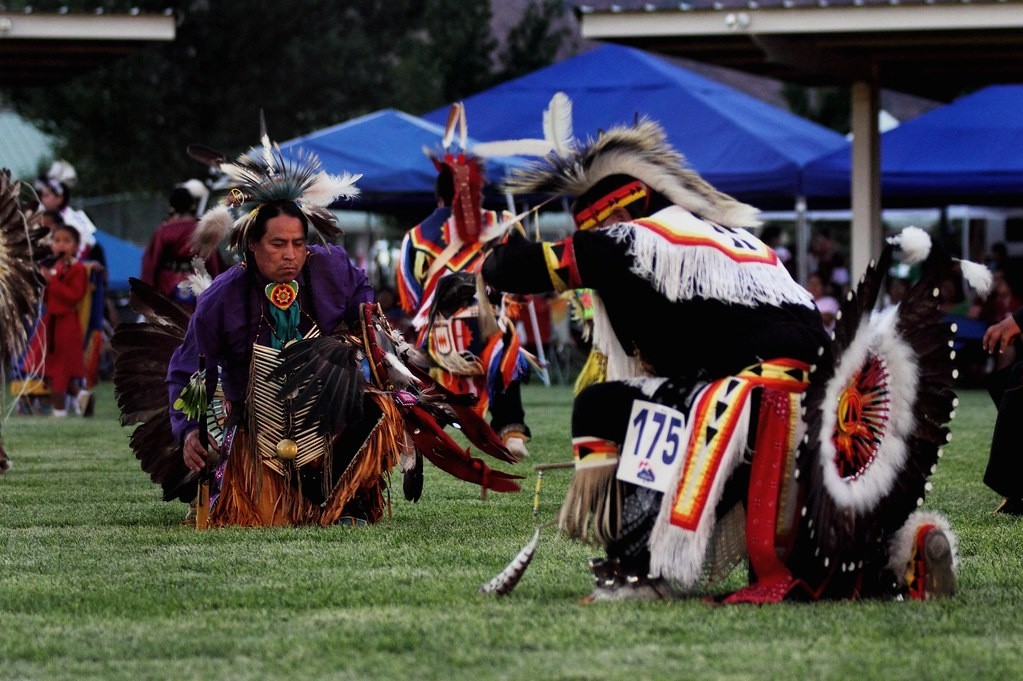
[969,238,1023,511]
[11,161,109,419]
[758,217,851,340]
[395,158,534,460]
[510,293,552,376]
[138,187,227,317]
[166,196,401,524]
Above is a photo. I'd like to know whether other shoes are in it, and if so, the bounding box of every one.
[582,584,675,602]
[505,439,532,458]
[906,522,959,600]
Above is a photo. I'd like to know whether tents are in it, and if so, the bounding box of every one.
[419,45,854,204]
[480,149,959,609]
[242,107,527,195]
[798,86,1023,211]
